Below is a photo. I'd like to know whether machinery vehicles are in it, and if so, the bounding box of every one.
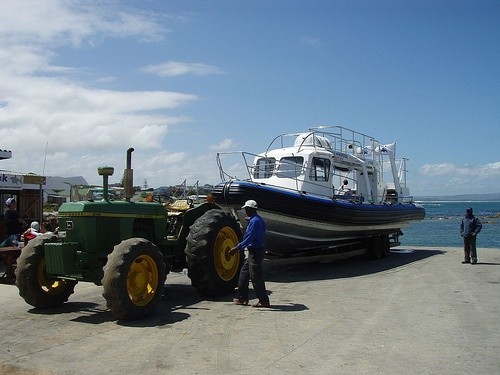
[14,147,244,321]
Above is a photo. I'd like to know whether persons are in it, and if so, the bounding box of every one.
[228,200,270,308]
[3,197,24,282]
[460,207,482,265]
[14,215,59,264]
[337,179,351,195]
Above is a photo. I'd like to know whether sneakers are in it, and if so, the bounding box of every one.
[253,302,270,307]
[234,300,248,305]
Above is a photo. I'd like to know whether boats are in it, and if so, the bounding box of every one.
[211,125,426,243]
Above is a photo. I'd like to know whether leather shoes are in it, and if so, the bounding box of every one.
[471,261,477,265]
[461,261,471,264]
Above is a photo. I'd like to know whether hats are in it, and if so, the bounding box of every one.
[241,200,258,208]
[466,207,473,211]
[31,221,39,232]
[6,197,15,205]
[343,179,348,184]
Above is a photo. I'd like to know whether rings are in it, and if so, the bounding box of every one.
[229,251,230,252]
